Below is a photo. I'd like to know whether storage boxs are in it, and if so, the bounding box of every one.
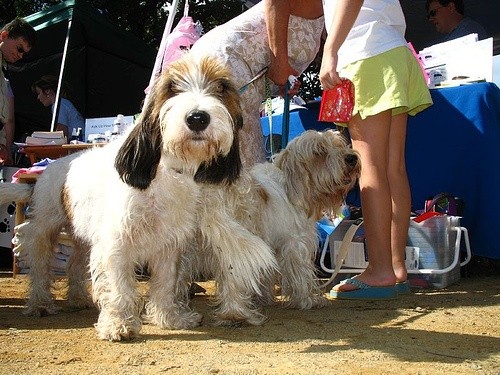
[329,216,463,288]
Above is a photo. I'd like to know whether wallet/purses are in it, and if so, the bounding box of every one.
[317,78,354,124]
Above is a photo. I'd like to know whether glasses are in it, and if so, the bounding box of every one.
[426,7,443,20]
[17,47,28,57]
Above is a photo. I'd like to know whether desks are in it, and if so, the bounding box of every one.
[19,143,104,166]
[260,82,500,266]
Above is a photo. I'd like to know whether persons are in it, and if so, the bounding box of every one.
[318,0,434,300]
[0,18,37,273]
[427,0,486,42]
[191,0,325,164]
[31,75,85,144]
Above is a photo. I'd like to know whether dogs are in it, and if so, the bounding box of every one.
[13,55,242,344]
[155,129,362,308]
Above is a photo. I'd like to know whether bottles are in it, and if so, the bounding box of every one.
[88,114,125,149]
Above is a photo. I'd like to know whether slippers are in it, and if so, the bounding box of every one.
[330,279,397,300]
[396,281,411,295]
